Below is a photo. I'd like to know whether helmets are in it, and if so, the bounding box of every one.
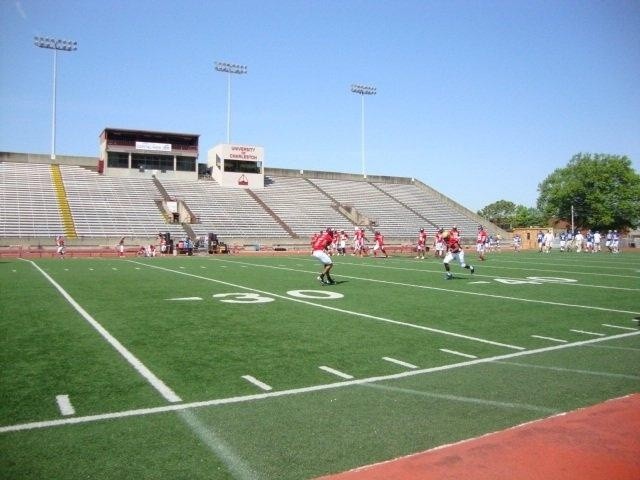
[441,230,451,240]
[326,226,339,234]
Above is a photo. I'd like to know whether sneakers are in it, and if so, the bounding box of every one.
[443,273,455,281]
[469,264,475,275]
[316,276,326,286]
[326,279,338,285]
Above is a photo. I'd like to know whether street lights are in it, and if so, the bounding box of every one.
[34,35,79,162]
[214,59,248,143]
[349,82,378,179]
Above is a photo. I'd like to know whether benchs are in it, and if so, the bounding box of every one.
[1,154,505,247]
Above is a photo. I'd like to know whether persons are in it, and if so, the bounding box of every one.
[414,225,474,280]
[119,233,193,258]
[310,227,389,285]
[477,225,503,261]
[513,228,618,256]
[56,236,64,259]
